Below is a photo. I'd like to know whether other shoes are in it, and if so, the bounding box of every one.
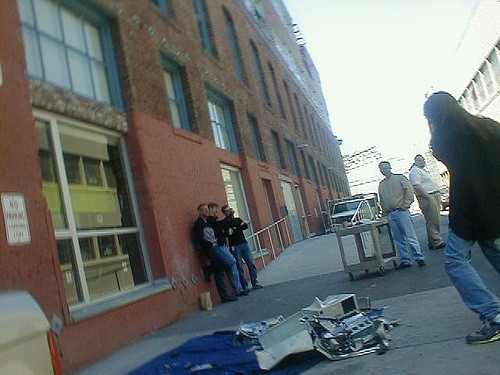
[223,296,239,303]
[236,291,248,296]
[429,242,446,249]
[396,263,412,270]
[243,287,249,292]
[252,284,263,289]
[417,259,425,266]
[201,266,211,282]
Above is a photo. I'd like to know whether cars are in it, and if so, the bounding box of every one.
[330,192,379,233]
[440,187,450,211]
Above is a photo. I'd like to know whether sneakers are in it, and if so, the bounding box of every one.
[466,314,500,344]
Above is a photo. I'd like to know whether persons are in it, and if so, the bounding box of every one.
[410,155,446,249]
[424,91,500,343]
[193,202,262,302]
[378,161,425,268]
